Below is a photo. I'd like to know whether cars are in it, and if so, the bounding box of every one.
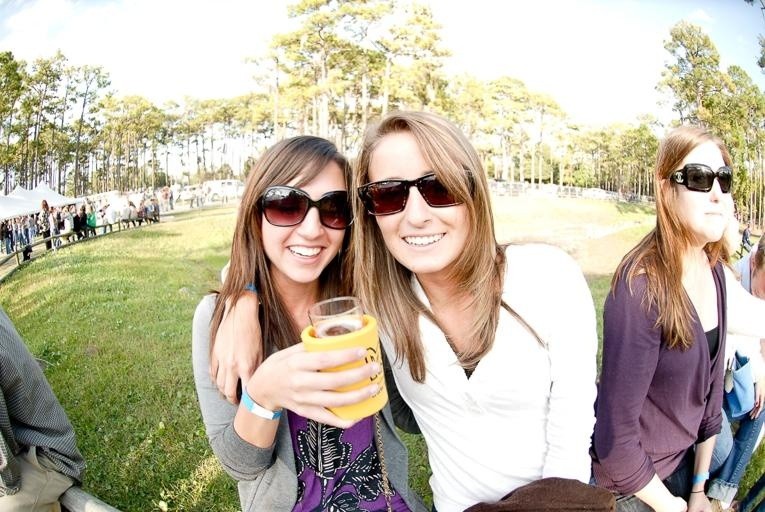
[181,185,201,200]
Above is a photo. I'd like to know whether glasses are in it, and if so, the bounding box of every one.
[258,184,352,231]
[668,162,733,195]
[356,170,471,217]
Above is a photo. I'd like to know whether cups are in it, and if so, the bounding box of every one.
[299,294,391,422]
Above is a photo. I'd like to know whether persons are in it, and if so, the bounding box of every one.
[191,181,213,207]
[209,106,598,512]
[0,183,178,257]
[0,300,84,512]
[742,226,761,250]
[700,236,764,510]
[591,118,738,512]
[190,135,431,512]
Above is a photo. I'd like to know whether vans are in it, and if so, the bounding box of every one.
[201,179,245,200]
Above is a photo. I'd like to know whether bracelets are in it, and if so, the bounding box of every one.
[238,393,285,420]
[693,471,712,486]
[690,489,705,496]
[242,282,259,294]
